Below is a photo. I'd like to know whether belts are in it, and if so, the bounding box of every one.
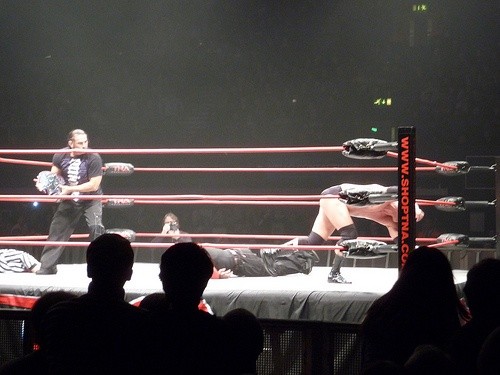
[227,248,239,268]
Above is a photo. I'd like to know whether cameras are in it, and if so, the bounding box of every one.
[170,222,179,231]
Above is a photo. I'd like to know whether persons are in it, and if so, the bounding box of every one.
[31,130,105,273]
[0,183,500,375]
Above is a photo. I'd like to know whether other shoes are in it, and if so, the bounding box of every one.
[36,266,57,274]
[310,250,320,263]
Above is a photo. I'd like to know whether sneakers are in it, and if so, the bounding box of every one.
[260,248,278,277]
[328,272,352,284]
